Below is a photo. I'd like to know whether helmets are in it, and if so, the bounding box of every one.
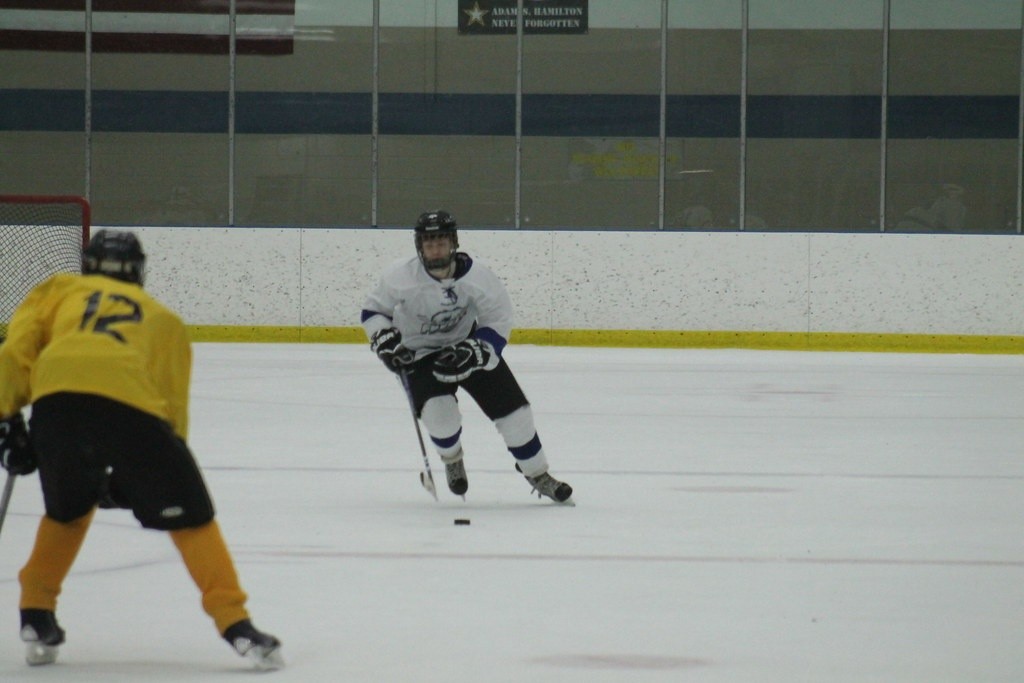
[81,228,145,284]
[414,211,459,270]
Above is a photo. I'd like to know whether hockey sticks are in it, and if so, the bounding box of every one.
[0,470,17,530]
[396,370,442,500]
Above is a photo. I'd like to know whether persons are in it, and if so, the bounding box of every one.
[1,227,285,672]
[361,208,577,510]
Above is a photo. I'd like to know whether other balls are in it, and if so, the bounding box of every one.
[454,518,470,526]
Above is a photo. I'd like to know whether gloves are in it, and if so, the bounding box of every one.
[96,472,131,509]
[0,413,36,475]
[434,338,490,385]
[371,327,415,378]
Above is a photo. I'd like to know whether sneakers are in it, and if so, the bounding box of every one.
[222,617,287,669]
[445,458,468,502]
[515,462,574,505]
[18,609,66,665]
